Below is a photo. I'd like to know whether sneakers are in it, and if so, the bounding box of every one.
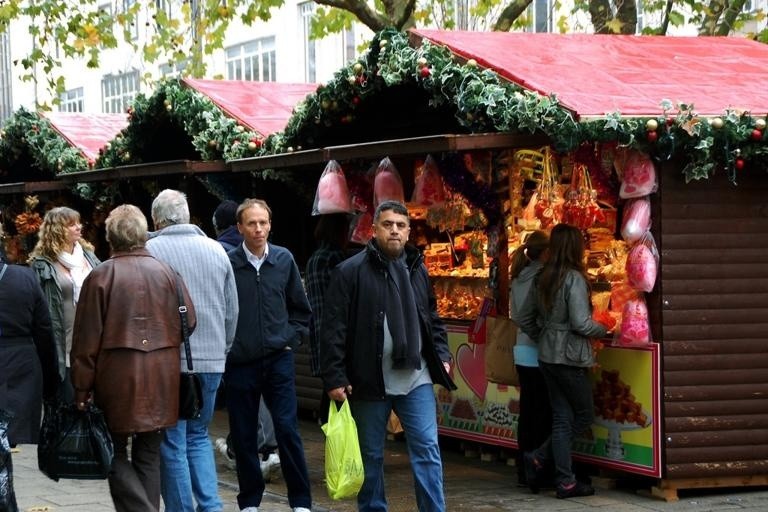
[215,438,236,470]
[257,454,282,481]
[556,481,594,499]
[524,453,541,493]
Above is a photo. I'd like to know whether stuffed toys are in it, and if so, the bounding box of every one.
[374,172,405,206]
[619,300,649,348]
[625,244,657,293]
[620,199,651,244]
[415,166,445,207]
[620,155,656,199]
[318,172,349,214]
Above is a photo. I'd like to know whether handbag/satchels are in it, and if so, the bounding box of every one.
[37,402,113,482]
[178,372,202,420]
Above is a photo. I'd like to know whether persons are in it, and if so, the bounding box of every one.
[0,189,319,512]
[517,223,608,499]
[506,230,558,492]
[319,199,459,511]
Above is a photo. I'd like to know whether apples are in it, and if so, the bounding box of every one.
[209,135,262,152]
[320,39,430,124]
[467,58,476,69]
[646,114,767,171]
